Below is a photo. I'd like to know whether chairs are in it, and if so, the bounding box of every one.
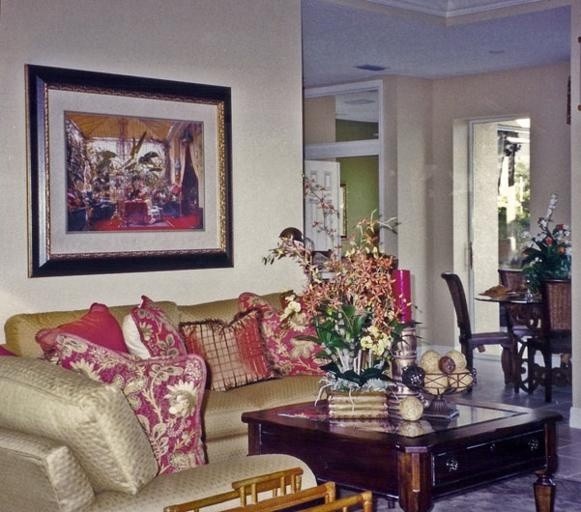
[441,269,572,403]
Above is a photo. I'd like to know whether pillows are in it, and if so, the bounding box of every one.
[34,295,210,478]
[178,292,326,392]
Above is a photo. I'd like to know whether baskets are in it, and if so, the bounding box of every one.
[402,365,477,396]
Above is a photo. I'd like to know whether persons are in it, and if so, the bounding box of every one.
[127,175,145,199]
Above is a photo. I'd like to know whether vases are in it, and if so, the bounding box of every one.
[328,393,389,418]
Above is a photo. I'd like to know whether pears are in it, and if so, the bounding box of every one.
[420,349,474,395]
[400,396,424,421]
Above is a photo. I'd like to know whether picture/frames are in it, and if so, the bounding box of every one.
[24,65,235,278]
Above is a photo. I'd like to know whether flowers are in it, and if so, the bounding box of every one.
[519,193,572,294]
[259,167,430,411]
